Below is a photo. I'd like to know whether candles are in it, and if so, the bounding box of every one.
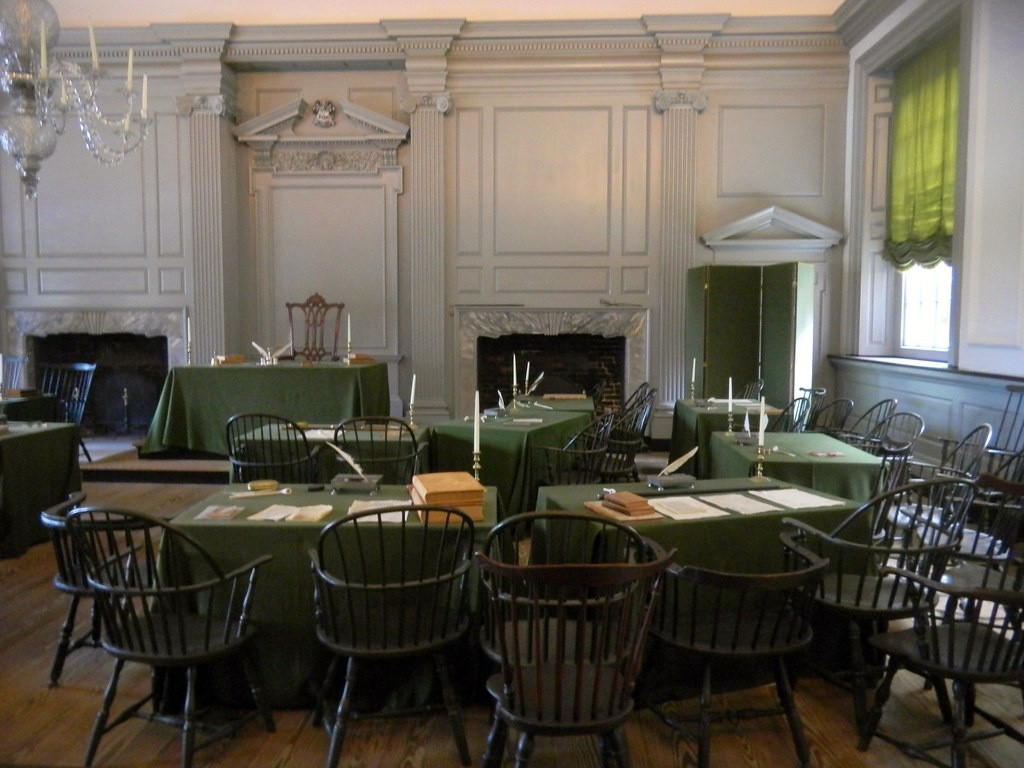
[728,376,732,412]
[127,47,134,91]
[86,17,98,69]
[347,314,351,342]
[512,353,518,386]
[187,317,191,348]
[473,389,481,452]
[759,395,766,433]
[141,74,149,117]
[691,357,696,382]
[40,15,47,71]
[526,361,529,381]
[410,373,416,405]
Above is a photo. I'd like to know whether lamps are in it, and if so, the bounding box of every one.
[1,1,154,203]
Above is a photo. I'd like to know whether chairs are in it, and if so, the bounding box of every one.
[330,415,428,485]
[586,398,655,484]
[939,426,989,513]
[861,411,924,507]
[286,293,343,360]
[985,384,1024,482]
[37,483,1024,768]
[43,361,94,463]
[845,399,894,451]
[625,383,649,411]
[535,412,612,488]
[222,411,321,484]
[808,398,855,436]
[773,397,810,432]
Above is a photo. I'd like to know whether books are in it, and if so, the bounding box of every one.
[544,393,587,398]
[6,387,36,396]
[410,471,485,523]
[584,490,663,522]
[211,353,243,365]
[343,352,375,363]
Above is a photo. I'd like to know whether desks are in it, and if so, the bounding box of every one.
[225,424,429,483]
[667,398,789,479]
[431,406,591,512]
[0,421,79,561]
[519,393,596,419]
[141,358,391,454]
